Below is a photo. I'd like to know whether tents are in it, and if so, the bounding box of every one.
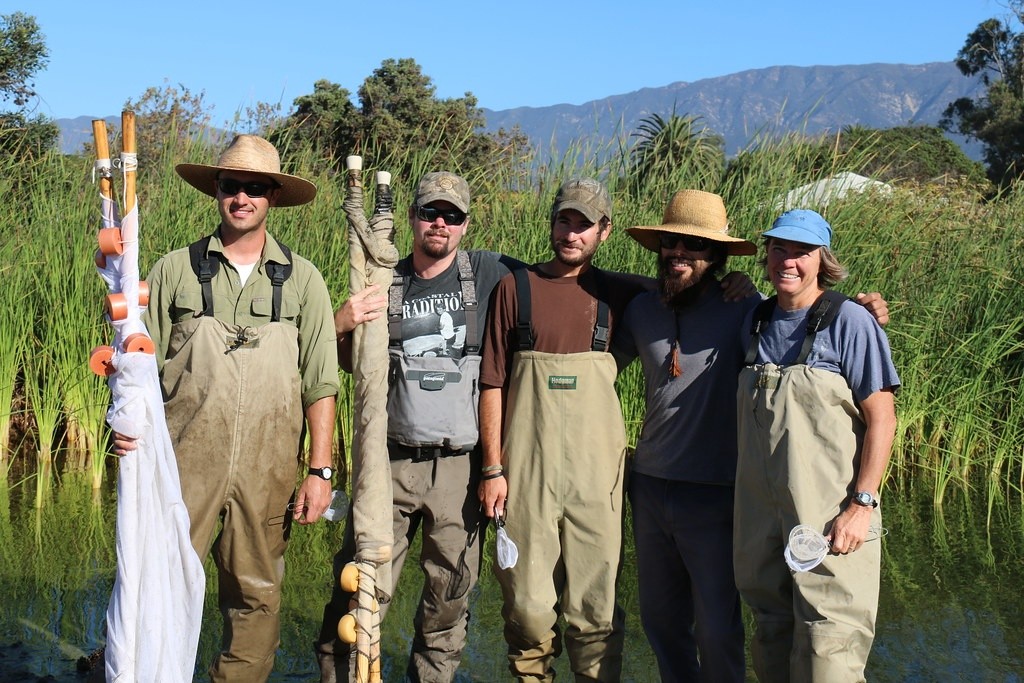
[755,171,893,212]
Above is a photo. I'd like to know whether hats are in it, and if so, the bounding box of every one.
[761,209,832,247]
[413,171,469,214]
[553,178,612,224]
[175,134,317,207]
[624,189,757,256]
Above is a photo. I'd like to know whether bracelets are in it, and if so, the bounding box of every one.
[481,464,504,480]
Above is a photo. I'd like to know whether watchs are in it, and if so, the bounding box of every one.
[852,491,878,509]
[308,467,332,479]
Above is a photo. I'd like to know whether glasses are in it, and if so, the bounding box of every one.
[659,235,710,251]
[414,205,466,226]
[216,178,274,198]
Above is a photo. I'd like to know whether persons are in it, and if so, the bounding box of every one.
[112,134,901,683]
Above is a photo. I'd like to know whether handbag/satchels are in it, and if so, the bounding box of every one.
[387,355,482,455]
